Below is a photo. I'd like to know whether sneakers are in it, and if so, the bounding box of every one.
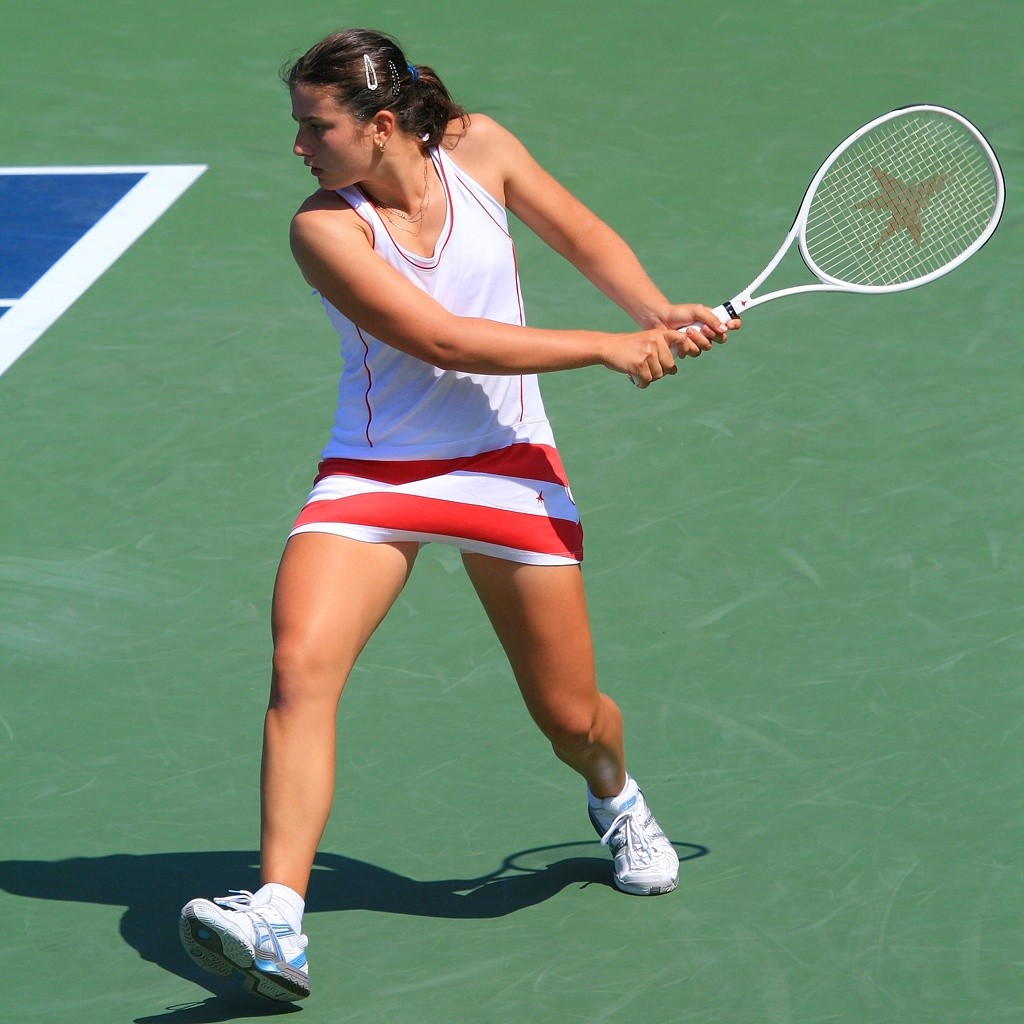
[177,894,311,1007]
[587,773,680,897]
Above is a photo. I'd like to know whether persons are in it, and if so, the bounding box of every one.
[178,30,742,1006]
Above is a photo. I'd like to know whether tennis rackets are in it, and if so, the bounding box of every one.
[626,104,1007,384]
